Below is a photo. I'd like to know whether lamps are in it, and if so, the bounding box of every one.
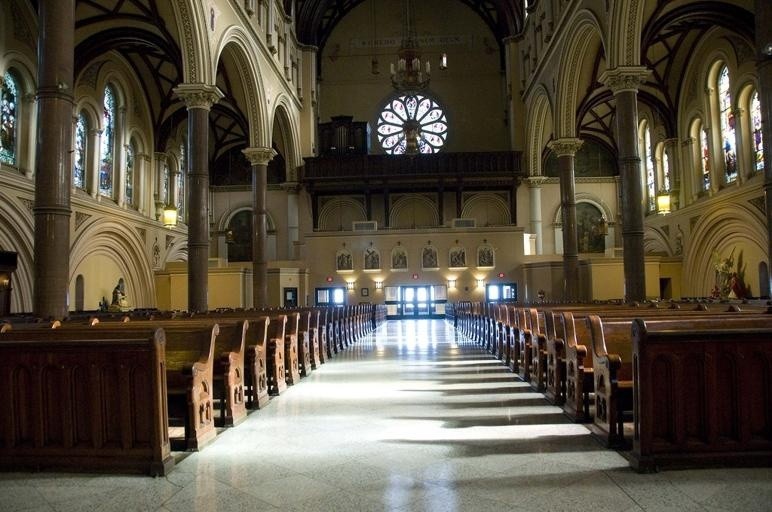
[388,1,431,100]
[438,0,448,70]
[370,1,380,76]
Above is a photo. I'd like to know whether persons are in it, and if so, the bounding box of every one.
[711,285,720,298]
[112,284,127,305]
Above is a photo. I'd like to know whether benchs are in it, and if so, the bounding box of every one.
[190,304,389,376]
[628,317,771,473]
[0,312,176,479]
[541,299,771,446]
[29,306,299,451]
[446,297,700,393]
